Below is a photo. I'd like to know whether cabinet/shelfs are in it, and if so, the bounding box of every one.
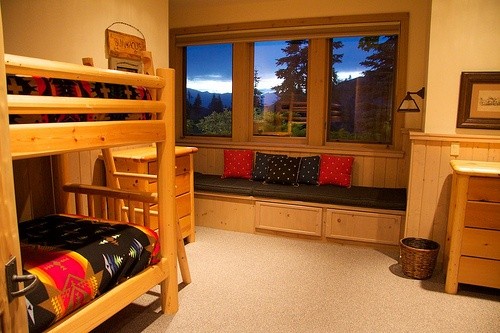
[442,159,500,295]
[98,146,199,243]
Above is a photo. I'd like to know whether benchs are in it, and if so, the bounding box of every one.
[175,143,407,246]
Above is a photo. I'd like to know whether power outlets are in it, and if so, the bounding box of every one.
[451,142,459,156]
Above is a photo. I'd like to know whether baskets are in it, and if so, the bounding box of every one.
[399,236,441,279]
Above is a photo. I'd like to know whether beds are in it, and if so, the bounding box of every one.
[0,14,179,333]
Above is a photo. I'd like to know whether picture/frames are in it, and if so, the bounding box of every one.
[456,71,500,130]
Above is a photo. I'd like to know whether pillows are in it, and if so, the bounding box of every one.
[220,149,255,180]
[252,152,288,182]
[317,154,354,189]
[266,157,303,187]
[298,156,320,186]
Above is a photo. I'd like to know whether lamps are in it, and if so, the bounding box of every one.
[397,88,424,113]
